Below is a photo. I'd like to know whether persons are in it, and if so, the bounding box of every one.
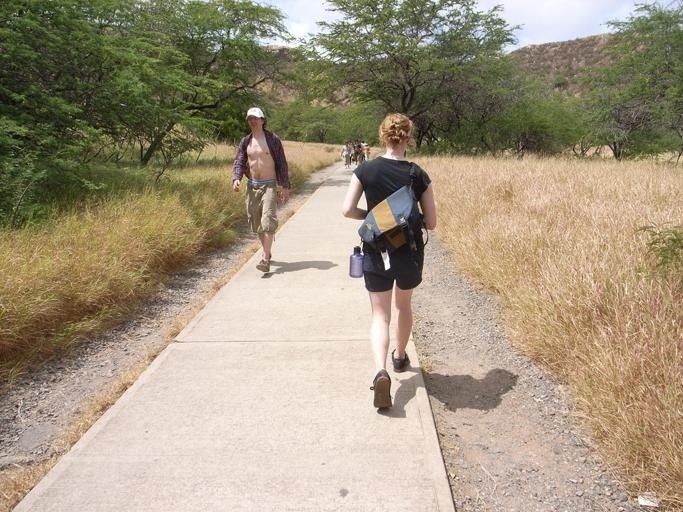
[340,139,370,169]
[232,107,290,272]
[342,112,437,408]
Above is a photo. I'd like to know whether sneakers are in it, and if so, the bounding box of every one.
[256,260,270,273]
[369,369,392,408]
[392,349,410,372]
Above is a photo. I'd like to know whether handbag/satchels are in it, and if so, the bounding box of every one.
[358,185,421,254]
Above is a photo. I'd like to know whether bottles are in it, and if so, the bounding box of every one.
[348,246,363,277]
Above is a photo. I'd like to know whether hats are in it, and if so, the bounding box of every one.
[245,106,264,120]
